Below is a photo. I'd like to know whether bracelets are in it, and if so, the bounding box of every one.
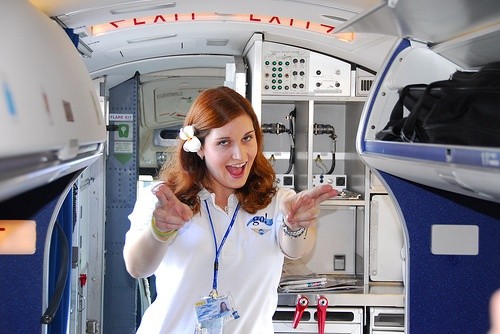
[282,220,308,238]
[151,217,176,237]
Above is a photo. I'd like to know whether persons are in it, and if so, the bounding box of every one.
[123,87,338,334]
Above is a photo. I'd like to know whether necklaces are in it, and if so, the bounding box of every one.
[216,199,228,211]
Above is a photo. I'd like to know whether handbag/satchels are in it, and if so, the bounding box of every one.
[375,61,500,147]
